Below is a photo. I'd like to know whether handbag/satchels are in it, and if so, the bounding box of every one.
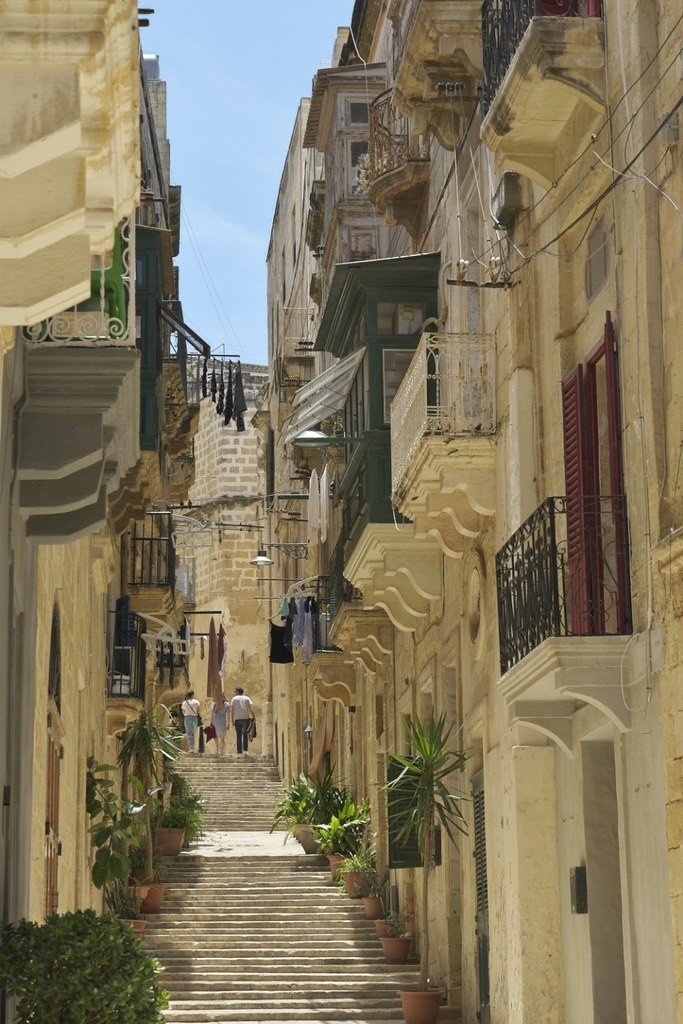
[205,723,216,743]
[197,713,203,727]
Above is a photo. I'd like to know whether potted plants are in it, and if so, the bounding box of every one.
[86,705,210,939]
[268,705,473,1024]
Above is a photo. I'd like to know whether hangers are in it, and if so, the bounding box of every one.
[266,596,296,622]
[200,611,222,640]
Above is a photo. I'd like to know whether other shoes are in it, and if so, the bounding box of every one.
[246,719,257,742]
[216,750,220,754]
[243,751,249,755]
[193,748,198,753]
[188,748,194,752]
[235,754,241,759]
[220,754,225,757]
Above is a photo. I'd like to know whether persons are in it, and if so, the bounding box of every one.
[181,690,201,753]
[210,695,231,758]
[231,687,255,756]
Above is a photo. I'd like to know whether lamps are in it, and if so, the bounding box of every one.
[291,431,365,448]
[249,543,310,566]
